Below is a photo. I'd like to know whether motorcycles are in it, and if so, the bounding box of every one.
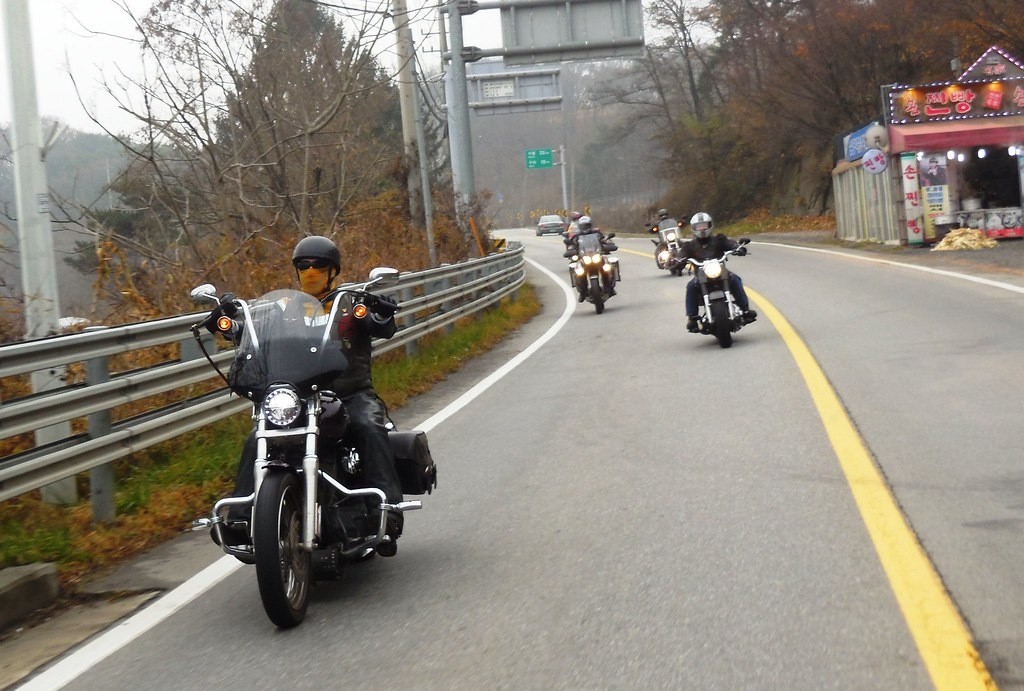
[659,236,756,347]
[643,214,690,276]
[189,262,442,627]
[558,229,621,313]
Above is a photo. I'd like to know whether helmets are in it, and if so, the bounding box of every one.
[571,211,583,222]
[658,208,669,221]
[690,213,714,238]
[292,236,341,271]
[578,216,592,233]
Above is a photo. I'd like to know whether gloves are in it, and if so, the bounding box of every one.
[203,307,235,334]
[370,294,397,318]
[671,260,682,270]
[737,247,747,256]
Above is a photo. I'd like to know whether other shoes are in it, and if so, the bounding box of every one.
[578,291,588,302]
[742,310,757,319]
[686,316,698,329]
[610,288,617,295]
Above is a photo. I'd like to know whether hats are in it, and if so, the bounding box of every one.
[929,158,938,163]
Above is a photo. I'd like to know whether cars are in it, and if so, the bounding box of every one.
[536,215,564,236]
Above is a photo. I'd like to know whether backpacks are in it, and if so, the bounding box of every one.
[388,430,437,494]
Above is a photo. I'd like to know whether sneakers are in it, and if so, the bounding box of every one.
[210,522,255,565]
[365,514,398,557]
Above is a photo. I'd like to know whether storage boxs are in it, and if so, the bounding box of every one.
[386,429,437,496]
[569,262,577,287]
[608,257,621,281]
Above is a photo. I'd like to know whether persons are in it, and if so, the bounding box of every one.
[650,208,686,234]
[568,211,582,234]
[210,237,404,555]
[564,216,617,258]
[926,158,946,184]
[672,212,756,330]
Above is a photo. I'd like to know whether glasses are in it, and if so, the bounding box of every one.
[295,259,332,271]
